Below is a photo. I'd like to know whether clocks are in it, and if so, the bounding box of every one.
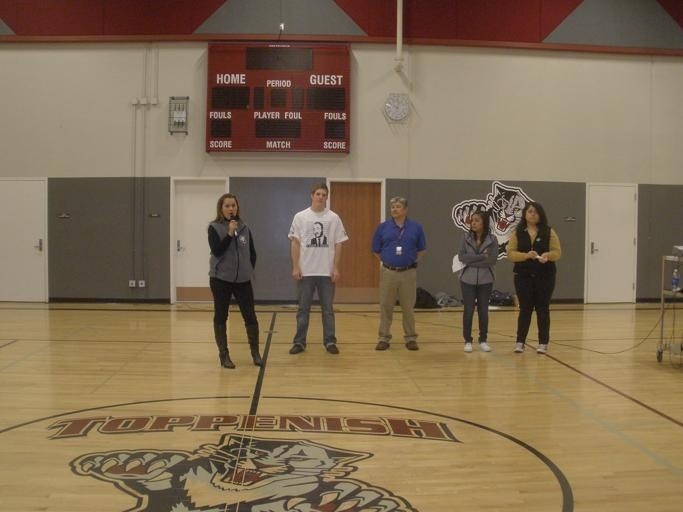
[384,94,410,121]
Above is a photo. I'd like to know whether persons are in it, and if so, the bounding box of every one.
[371,196,426,350]
[287,183,349,354]
[457,211,498,352]
[507,201,562,353]
[208,193,263,368]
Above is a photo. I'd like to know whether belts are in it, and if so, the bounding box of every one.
[382,262,415,271]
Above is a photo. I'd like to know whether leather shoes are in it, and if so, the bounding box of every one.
[289,344,303,354]
[406,341,418,350]
[375,341,390,350]
[325,344,339,354]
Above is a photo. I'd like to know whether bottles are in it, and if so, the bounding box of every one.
[672,268,681,292]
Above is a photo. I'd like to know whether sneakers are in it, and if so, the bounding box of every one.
[478,342,491,352]
[513,342,524,353]
[463,343,471,352]
[536,344,547,353]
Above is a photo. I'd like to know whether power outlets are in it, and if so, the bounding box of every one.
[138,281,145,288]
[129,280,135,288]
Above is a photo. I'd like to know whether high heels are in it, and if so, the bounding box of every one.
[219,353,236,369]
[251,351,263,366]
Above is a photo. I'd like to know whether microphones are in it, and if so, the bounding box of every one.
[230,215,238,237]
